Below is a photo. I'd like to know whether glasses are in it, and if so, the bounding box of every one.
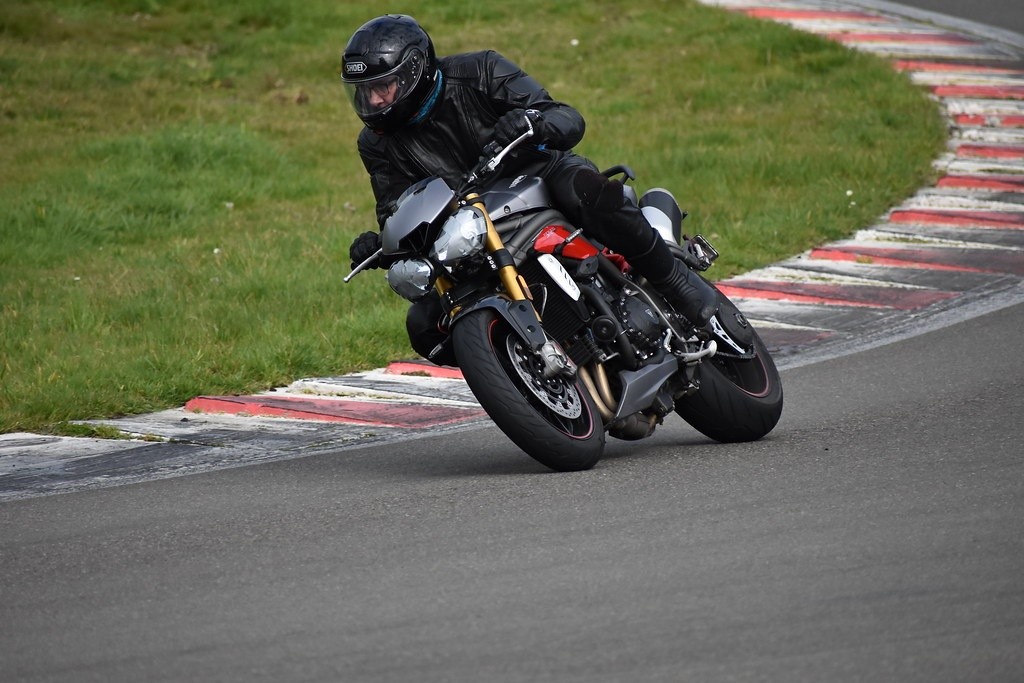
[355,77,397,101]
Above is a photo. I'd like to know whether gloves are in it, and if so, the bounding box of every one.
[493,108,541,158]
[349,230,381,271]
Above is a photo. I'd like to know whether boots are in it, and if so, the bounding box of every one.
[625,228,718,327]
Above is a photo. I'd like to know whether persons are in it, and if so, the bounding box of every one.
[339,15,719,368]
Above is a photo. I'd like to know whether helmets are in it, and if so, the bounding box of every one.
[341,14,436,134]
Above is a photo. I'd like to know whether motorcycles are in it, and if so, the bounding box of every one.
[339,104,785,470]
